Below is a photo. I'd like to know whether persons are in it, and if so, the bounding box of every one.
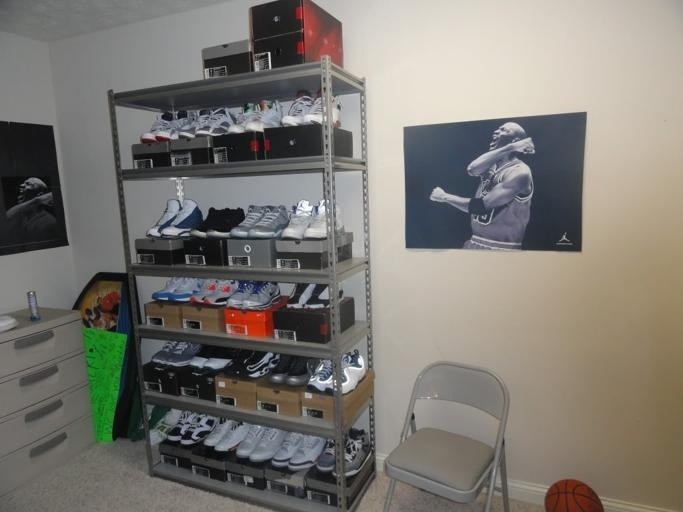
[429,121,535,253]
[8,177,56,243]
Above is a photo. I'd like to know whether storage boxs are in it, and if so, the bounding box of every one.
[0,307,96,500]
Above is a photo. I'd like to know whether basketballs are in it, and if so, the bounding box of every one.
[545,479,604,512]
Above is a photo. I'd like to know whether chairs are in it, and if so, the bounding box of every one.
[381,359,512,511]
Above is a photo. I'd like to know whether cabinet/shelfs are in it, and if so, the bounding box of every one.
[105,55,376,512]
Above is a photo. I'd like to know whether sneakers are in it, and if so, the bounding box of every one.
[287,435,327,471]
[204,351,233,371]
[332,428,371,478]
[214,422,251,451]
[166,343,201,367]
[152,277,183,301]
[196,109,228,137]
[167,410,198,441]
[225,350,253,377]
[304,199,343,240]
[307,359,336,393]
[162,200,203,238]
[178,110,212,139]
[248,101,283,132]
[230,205,274,239]
[228,101,256,133]
[241,281,282,310]
[171,278,204,302]
[139,116,167,143]
[325,350,366,396]
[190,280,217,303]
[151,341,179,367]
[158,112,188,140]
[227,281,257,309]
[249,427,287,462]
[208,207,245,237]
[283,97,313,129]
[189,346,209,371]
[146,199,182,237]
[235,425,266,459]
[204,280,240,305]
[180,414,220,445]
[248,204,289,239]
[203,419,238,446]
[270,353,292,385]
[191,208,224,238]
[316,438,335,472]
[286,283,316,310]
[305,94,341,125]
[303,283,344,310]
[286,356,316,388]
[281,199,313,239]
[271,432,305,468]
[240,352,280,380]
